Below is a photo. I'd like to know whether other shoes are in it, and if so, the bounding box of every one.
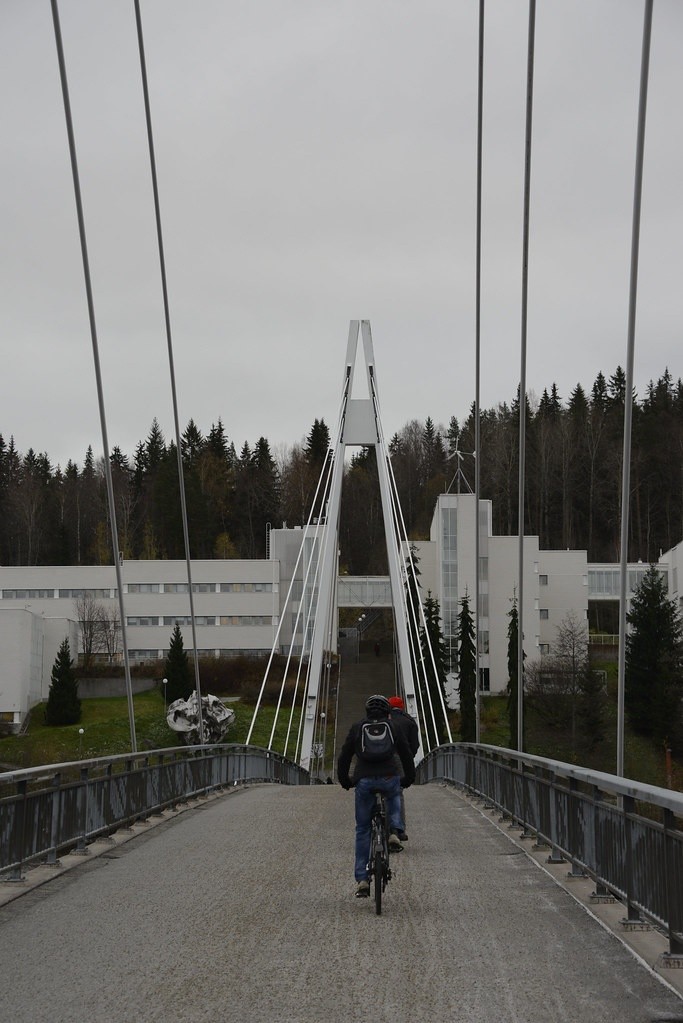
[388,834,400,853]
[398,832,409,841]
[355,880,369,895]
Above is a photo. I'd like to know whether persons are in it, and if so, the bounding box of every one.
[381,696,421,842]
[374,638,380,656]
[326,776,334,784]
[337,694,404,899]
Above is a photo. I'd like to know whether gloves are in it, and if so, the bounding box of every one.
[345,781,354,791]
[400,777,411,788]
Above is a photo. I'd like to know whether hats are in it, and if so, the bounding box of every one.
[388,697,404,709]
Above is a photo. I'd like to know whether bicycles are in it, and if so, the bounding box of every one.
[349,782,406,914]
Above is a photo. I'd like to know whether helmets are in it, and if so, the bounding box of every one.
[366,694,391,714]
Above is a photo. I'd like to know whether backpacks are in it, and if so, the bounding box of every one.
[355,719,394,763]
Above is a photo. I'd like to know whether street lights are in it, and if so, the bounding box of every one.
[320,712,325,731]
[163,679,168,717]
[359,618,362,642]
[362,614,365,641]
[79,728,84,760]
